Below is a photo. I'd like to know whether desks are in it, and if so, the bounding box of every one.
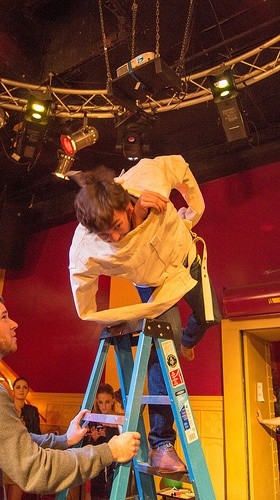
[157,488,196,500]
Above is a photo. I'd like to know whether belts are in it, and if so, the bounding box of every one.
[187,236,214,322]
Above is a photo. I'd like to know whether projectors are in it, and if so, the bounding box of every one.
[112,52,185,104]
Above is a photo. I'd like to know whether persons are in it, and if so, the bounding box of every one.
[84,384,132,500]
[4,376,41,500]
[256,408,280,426]
[67,154,221,475]
[1,296,141,494]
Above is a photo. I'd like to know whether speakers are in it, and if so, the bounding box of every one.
[0,202,33,270]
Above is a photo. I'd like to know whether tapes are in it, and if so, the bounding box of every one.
[179,494,186,497]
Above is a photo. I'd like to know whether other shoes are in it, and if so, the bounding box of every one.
[146,442,190,475]
[180,342,196,361]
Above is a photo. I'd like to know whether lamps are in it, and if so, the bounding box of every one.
[206,63,247,143]
[123,123,150,161]
[53,112,98,179]
[11,72,53,168]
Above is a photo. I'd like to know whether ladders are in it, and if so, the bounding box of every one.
[55,318,217,500]
